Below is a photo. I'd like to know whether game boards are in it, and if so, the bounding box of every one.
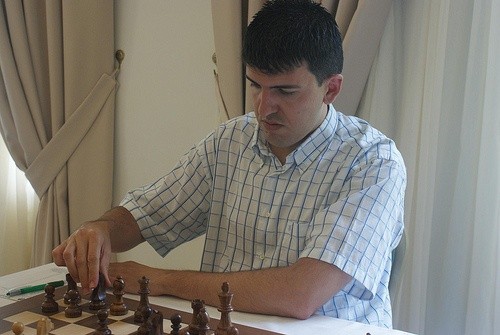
[0,285,281,335]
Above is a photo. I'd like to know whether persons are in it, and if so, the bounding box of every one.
[51,0,407,328]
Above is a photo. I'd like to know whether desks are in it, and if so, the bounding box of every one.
[1,262,414,335]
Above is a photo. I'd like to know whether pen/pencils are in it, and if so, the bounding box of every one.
[6,280,64,297]
